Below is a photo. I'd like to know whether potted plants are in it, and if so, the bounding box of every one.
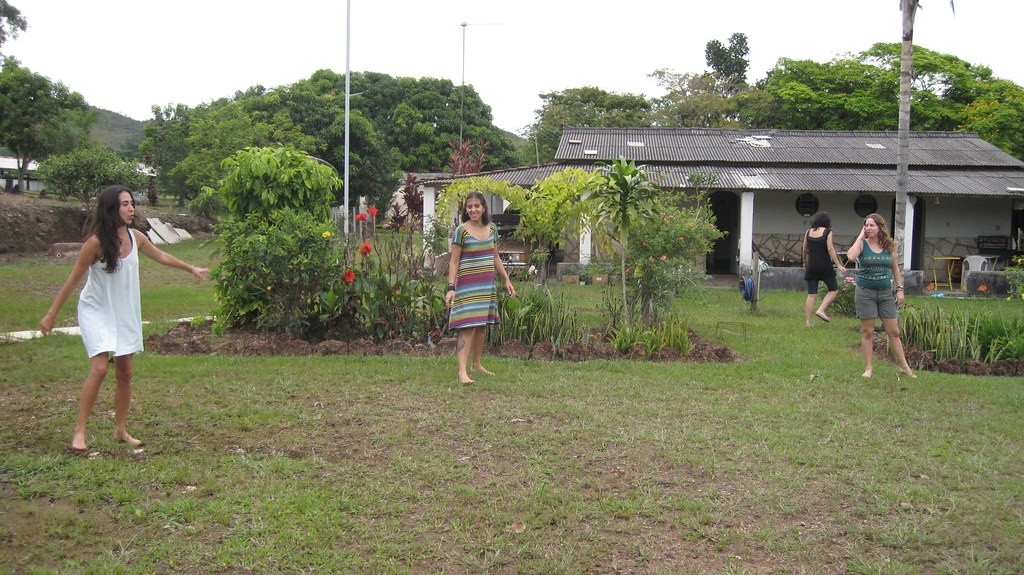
[562,263,619,285]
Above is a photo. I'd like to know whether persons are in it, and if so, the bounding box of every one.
[446,191,516,385]
[39,186,210,450]
[803,215,846,328]
[846,213,918,380]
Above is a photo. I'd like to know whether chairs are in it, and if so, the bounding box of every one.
[961,255,987,289]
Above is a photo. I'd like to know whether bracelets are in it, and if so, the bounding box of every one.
[897,285,904,291]
[448,283,455,291]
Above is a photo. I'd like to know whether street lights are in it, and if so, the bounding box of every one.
[458,19,471,173]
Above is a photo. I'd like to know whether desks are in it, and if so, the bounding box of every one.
[965,254,1001,271]
[774,260,802,267]
[927,255,960,291]
[836,251,850,268]
